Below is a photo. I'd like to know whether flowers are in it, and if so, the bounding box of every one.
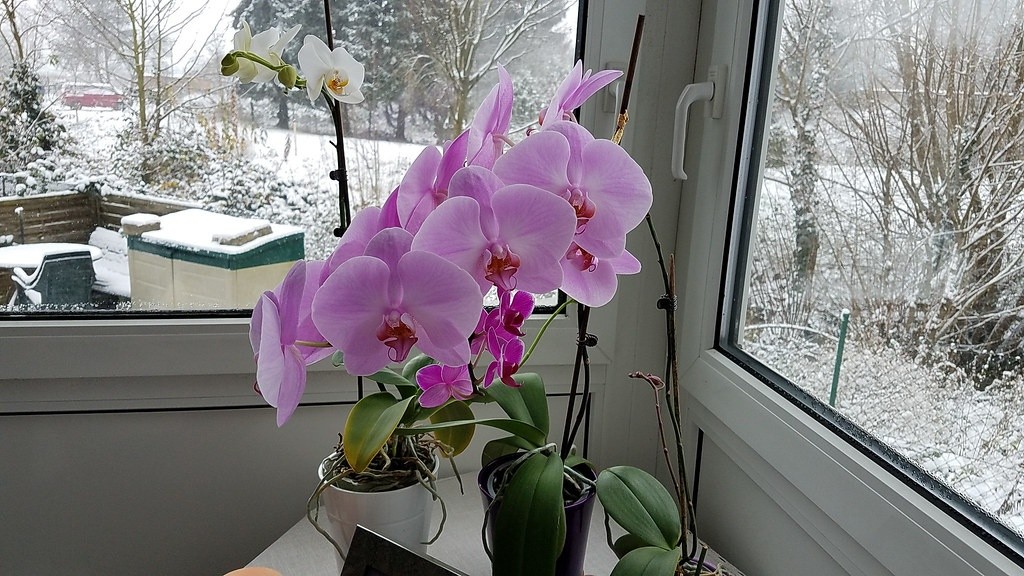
[246,59,655,576]
[216,20,478,565]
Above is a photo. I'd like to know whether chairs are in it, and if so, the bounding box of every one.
[11,249,98,311]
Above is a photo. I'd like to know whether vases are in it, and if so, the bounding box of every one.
[315,443,439,571]
[477,452,597,576]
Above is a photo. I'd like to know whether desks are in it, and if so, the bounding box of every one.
[0,243,104,277]
[243,470,744,576]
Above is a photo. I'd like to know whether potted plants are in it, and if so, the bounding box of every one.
[593,213,732,576]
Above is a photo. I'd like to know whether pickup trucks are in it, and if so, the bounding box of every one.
[59,81,134,112]
